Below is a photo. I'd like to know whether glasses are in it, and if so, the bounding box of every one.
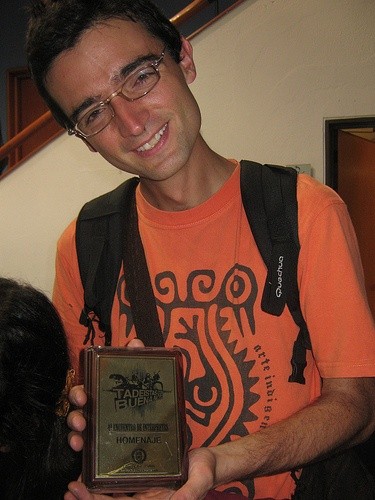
[66,39,169,138]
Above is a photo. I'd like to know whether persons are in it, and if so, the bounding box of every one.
[23,2,375,500]
